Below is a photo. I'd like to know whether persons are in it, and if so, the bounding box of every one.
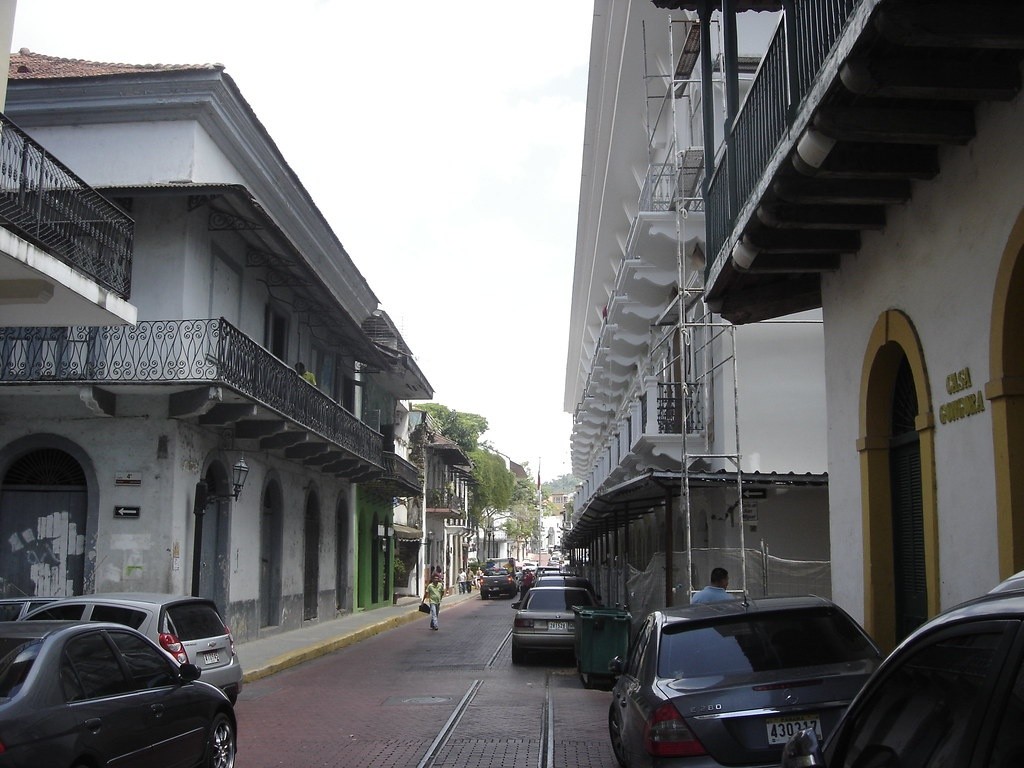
[456,568,467,594]
[466,568,476,593]
[295,361,317,387]
[422,575,445,631]
[476,567,482,589]
[519,570,533,601]
[431,566,444,580]
[690,568,735,605]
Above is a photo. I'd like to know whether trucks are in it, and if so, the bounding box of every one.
[486,557,519,581]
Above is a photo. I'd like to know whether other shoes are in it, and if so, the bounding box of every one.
[429,623,433,630]
[435,627,438,630]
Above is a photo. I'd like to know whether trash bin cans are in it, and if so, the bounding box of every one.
[570,603,633,692]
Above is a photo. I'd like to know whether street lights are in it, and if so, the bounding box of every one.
[191,456,250,601]
[415,529,435,597]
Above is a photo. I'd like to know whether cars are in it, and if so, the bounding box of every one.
[547,549,569,567]
[515,561,539,580]
[0,621,238,768]
[0,595,243,709]
[511,587,603,665]
[477,568,517,600]
[781,570,1024,768]
[530,566,602,603]
[606,594,885,767]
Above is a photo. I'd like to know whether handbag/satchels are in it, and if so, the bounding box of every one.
[419,603,430,614]
